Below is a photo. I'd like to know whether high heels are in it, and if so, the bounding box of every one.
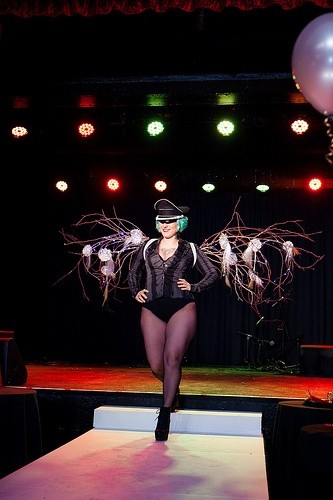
[174,387,180,408]
[154,407,172,440]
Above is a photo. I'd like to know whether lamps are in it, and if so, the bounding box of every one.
[11,111,325,199]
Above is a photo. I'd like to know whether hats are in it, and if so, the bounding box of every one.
[153,199,190,220]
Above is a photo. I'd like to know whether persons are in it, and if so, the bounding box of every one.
[128,198,217,441]
